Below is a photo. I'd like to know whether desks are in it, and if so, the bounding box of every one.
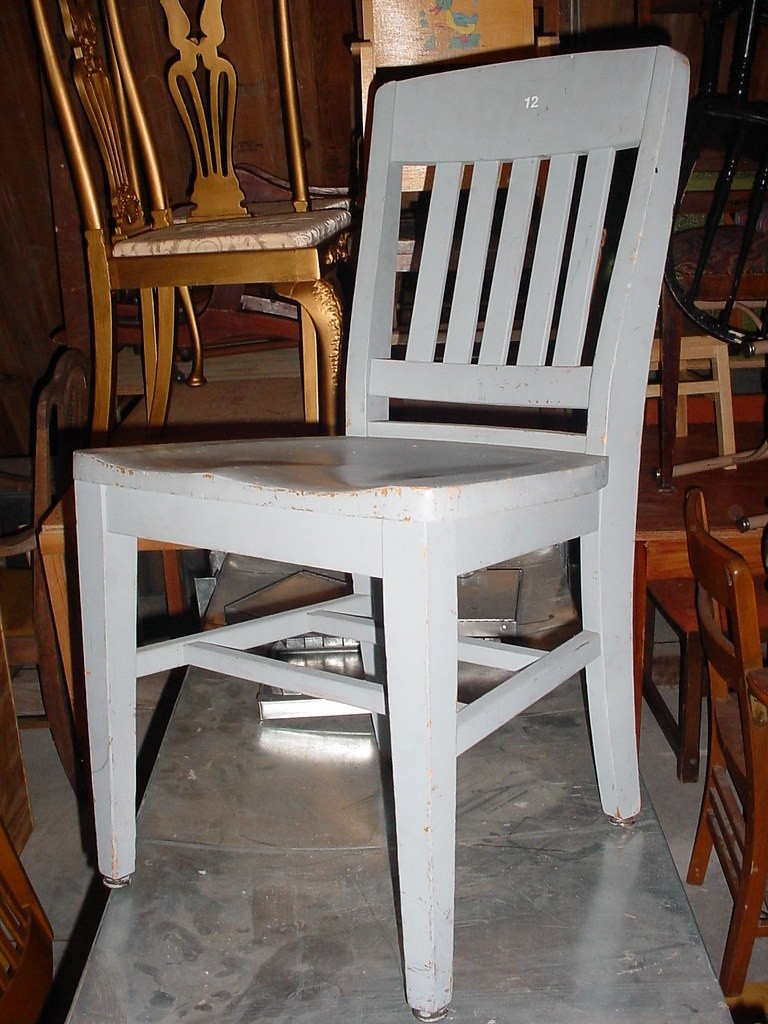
[38,378,572,740]
[62,543,734,1024]
[634,420,768,758]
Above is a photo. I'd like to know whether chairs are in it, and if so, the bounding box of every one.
[73,45,691,1022]
[685,489,768,998]
[658,150,768,492]
[0,817,54,1024]
[0,348,93,800]
[663,0,768,346]
[111,0,322,427]
[643,394,768,783]
[32,0,351,449]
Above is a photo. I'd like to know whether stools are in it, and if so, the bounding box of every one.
[645,336,737,471]
